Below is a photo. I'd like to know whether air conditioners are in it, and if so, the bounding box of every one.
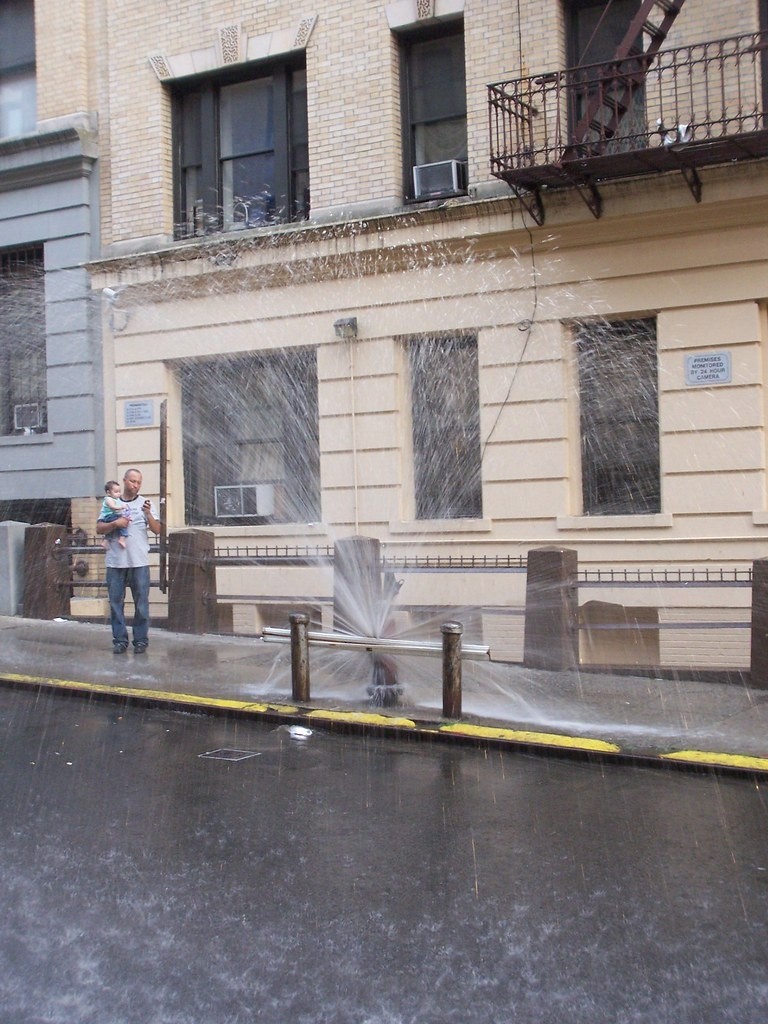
[413,160,464,198]
[213,484,275,518]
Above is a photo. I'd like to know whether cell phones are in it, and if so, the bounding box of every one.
[142,500,150,511]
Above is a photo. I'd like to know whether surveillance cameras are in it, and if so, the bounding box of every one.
[103,288,116,307]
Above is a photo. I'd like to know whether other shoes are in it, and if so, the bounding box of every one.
[134,643,146,654]
[114,642,127,653]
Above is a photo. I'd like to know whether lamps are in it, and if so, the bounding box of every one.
[335,318,358,338]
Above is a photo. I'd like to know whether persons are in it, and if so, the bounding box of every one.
[95,468,162,654]
[100,479,131,549]
